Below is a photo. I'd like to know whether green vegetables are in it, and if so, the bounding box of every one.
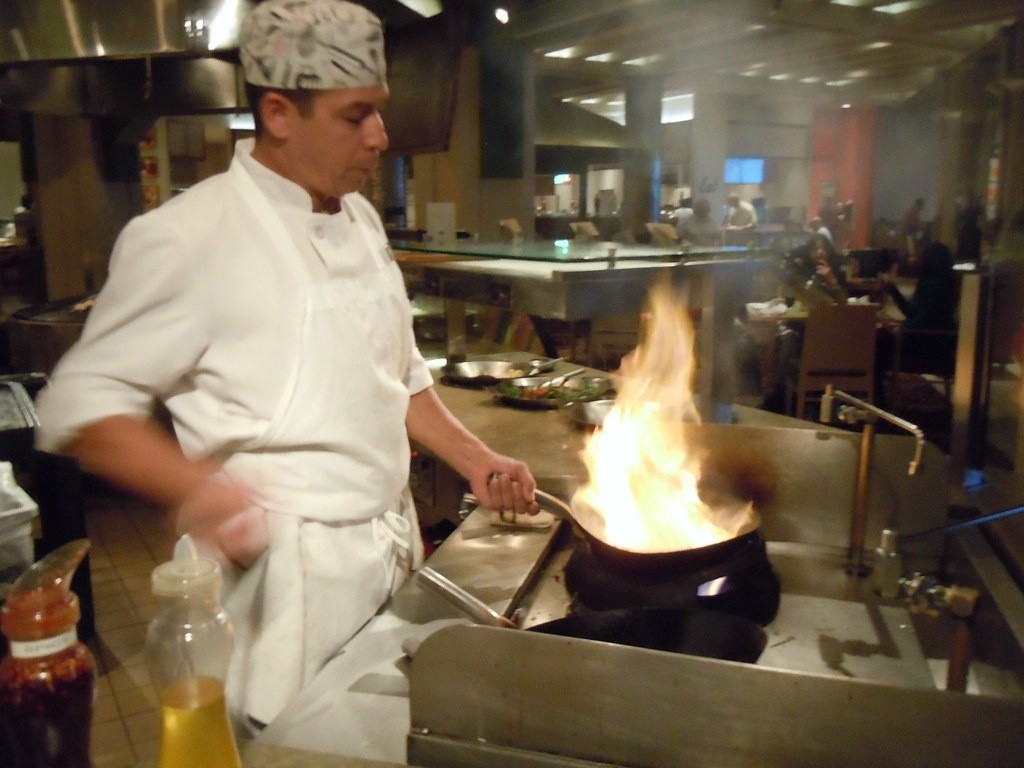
[495,383,600,404]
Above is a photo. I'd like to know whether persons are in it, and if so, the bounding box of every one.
[680,199,718,247]
[777,232,955,372]
[674,195,692,217]
[35,1,543,729]
[952,160,985,264]
[903,199,924,234]
[723,193,759,247]
[812,195,835,249]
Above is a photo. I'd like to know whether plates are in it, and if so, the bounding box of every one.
[745,302,787,315]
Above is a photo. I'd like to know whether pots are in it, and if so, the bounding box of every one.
[567,399,615,425]
[489,472,763,614]
[441,356,566,386]
[416,566,768,664]
[490,368,612,407]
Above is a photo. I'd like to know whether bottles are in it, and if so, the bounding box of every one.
[143,533,244,768]
[0,537,101,768]
[870,527,902,600]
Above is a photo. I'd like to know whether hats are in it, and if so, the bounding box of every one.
[239,0,386,90]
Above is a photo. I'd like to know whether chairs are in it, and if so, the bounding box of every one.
[785,303,882,416]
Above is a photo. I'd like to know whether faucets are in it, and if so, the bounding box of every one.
[818,381,926,478]
